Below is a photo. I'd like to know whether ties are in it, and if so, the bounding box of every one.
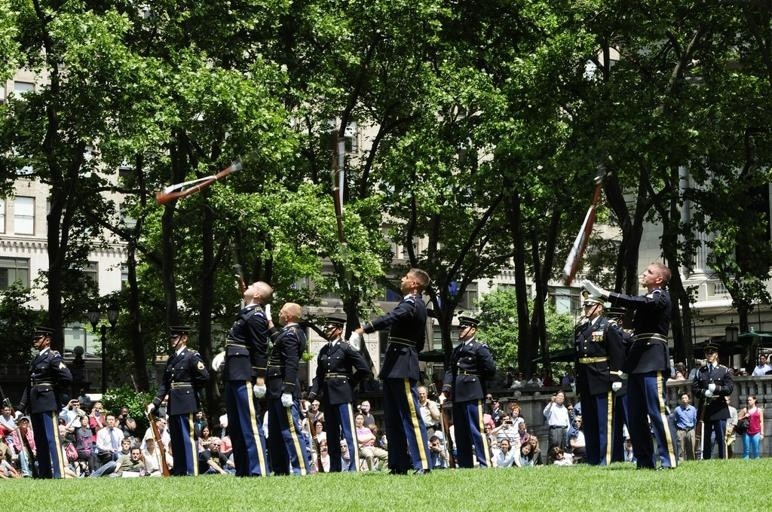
[110,428,117,449]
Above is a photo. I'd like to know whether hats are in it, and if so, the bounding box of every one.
[17,413,30,423]
[323,316,347,329]
[167,325,191,338]
[458,316,479,330]
[582,289,607,307]
[701,343,721,354]
[32,326,56,339]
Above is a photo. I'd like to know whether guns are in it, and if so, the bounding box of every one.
[295,382,324,473]
[564,176,602,284]
[232,262,247,300]
[131,375,170,477]
[1,392,38,477]
[156,163,242,201]
[426,374,455,468]
[331,137,345,240]
[694,378,713,452]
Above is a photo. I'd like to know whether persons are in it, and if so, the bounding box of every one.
[211,279,275,474]
[300,315,371,473]
[144,325,211,477]
[581,262,672,470]
[689,342,735,460]
[1,397,173,477]
[347,266,433,476]
[438,316,497,467]
[675,361,702,381]
[573,291,627,466]
[338,386,542,476]
[603,306,640,465]
[505,365,579,389]
[752,354,771,376]
[193,399,331,477]
[14,325,74,479]
[543,389,765,465]
[265,300,311,476]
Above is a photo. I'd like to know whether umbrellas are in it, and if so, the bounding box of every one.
[418,349,451,362]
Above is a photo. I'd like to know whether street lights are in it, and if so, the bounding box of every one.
[88,294,120,394]
[724,316,739,375]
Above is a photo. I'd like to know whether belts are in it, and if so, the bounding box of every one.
[578,355,610,365]
[323,372,352,383]
[29,377,56,387]
[223,337,253,350]
[386,335,418,349]
[267,359,287,369]
[631,331,669,345]
[169,381,194,390]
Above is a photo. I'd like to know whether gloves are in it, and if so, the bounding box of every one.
[264,302,273,321]
[13,410,25,424]
[281,393,295,408]
[211,351,227,371]
[349,331,362,352]
[705,383,716,398]
[611,381,623,392]
[582,279,603,298]
[252,384,267,399]
[145,402,156,417]
